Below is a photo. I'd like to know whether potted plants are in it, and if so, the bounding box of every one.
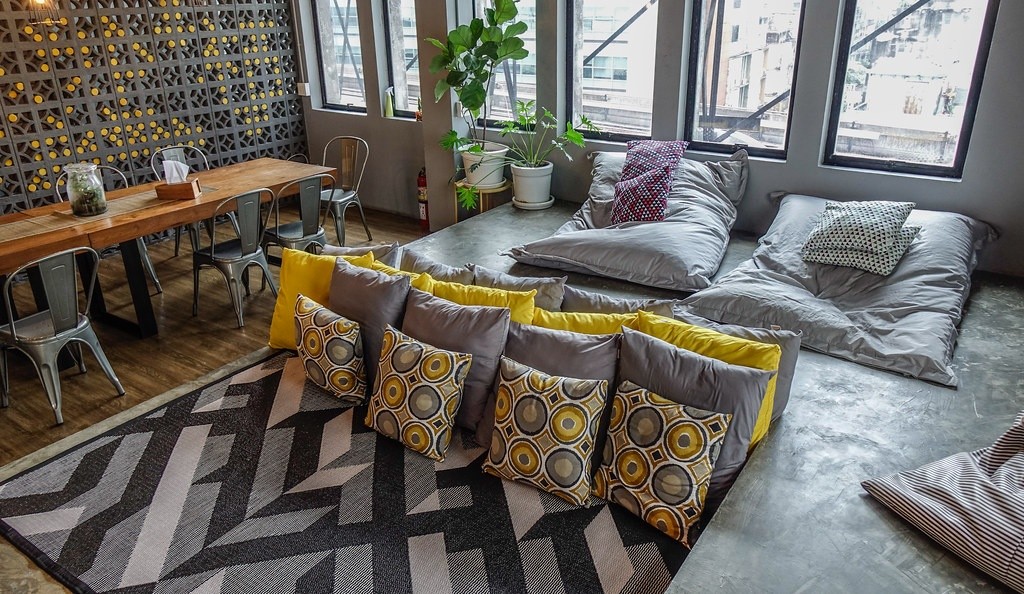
[423,0,603,211]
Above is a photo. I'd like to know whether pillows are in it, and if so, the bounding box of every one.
[269,140,922,554]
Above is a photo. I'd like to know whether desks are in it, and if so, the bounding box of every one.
[21,157,338,339]
[0,211,90,374]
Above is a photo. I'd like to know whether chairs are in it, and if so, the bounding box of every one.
[0,134,371,424]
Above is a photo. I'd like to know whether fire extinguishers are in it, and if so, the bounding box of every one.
[416,167,429,225]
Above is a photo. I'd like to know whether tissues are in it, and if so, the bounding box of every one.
[154,160,203,200]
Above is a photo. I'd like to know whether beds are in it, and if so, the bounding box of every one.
[396,198,1024,594]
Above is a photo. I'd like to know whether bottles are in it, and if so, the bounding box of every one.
[63,163,108,216]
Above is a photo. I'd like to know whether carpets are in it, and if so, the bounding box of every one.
[0,351,721,594]
